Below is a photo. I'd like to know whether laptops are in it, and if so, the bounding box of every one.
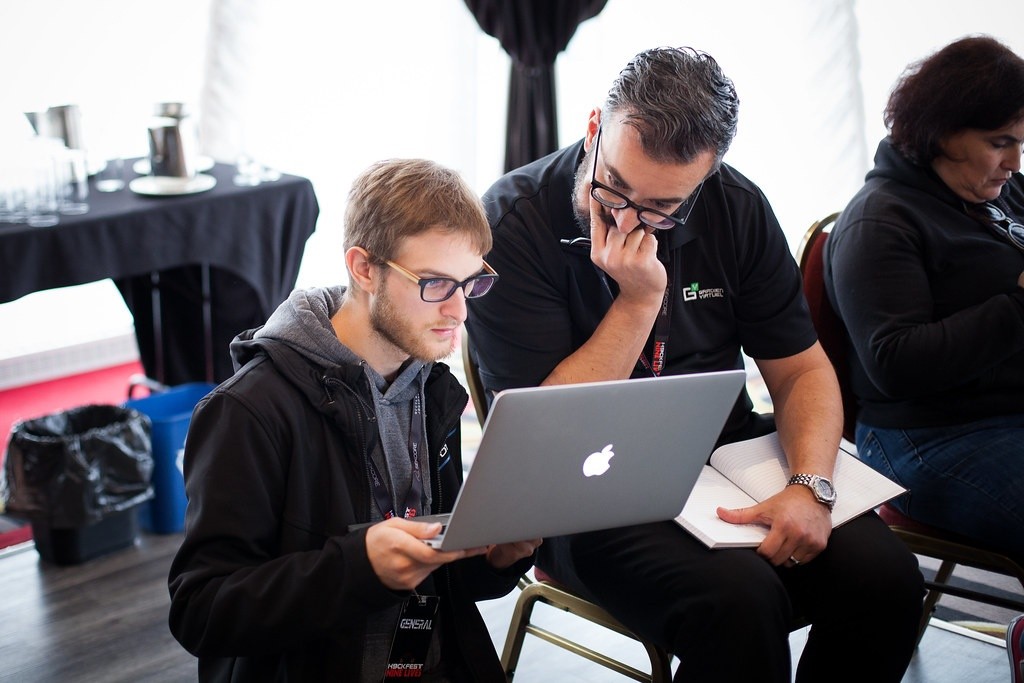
[349,369,746,551]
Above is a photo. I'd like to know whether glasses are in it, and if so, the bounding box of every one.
[366,249,498,303]
[591,124,704,229]
[964,201,1024,250]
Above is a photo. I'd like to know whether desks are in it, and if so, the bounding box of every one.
[0,156,319,384]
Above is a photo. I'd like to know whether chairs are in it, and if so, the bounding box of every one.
[461,324,675,683]
[793,212,1024,648]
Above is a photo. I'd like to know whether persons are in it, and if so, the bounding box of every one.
[818,37,1024,683]
[465,45,934,683]
[166,155,551,683]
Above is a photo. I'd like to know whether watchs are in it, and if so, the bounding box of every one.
[785,473,839,513]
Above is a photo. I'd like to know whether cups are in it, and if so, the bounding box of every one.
[1,136,89,227]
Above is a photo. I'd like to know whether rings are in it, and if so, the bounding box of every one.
[789,556,802,565]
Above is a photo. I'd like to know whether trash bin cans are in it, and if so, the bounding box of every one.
[15,405,140,566]
[124,381,217,534]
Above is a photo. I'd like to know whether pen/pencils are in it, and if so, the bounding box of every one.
[560,237,591,249]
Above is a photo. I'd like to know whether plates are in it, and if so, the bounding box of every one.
[130,155,217,196]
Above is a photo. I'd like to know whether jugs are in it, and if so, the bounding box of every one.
[146,102,187,181]
